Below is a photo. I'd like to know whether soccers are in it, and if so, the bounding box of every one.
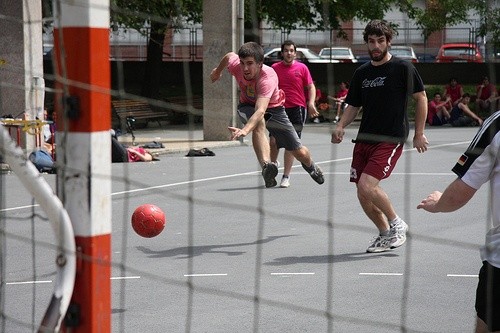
[132,204,167,238]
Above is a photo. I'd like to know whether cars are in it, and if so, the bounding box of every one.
[318,47,360,63]
[431,43,483,64]
[264,47,340,64]
[389,45,421,63]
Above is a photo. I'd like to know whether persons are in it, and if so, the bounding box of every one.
[270,40,319,187]
[209,42,324,187]
[304,80,323,123]
[42,107,58,144]
[425,77,500,127]
[334,80,349,123]
[111,137,155,162]
[419,111,500,332]
[30,142,56,175]
[330,20,428,252]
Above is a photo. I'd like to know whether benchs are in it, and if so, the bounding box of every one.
[112,100,169,146]
[165,95,204,123]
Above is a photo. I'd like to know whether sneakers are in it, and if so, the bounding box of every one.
[387,221,408,249]
[280,177,290,187]
[262,162,278,187]
[367,235,390,252]
[302,162,324,185]
